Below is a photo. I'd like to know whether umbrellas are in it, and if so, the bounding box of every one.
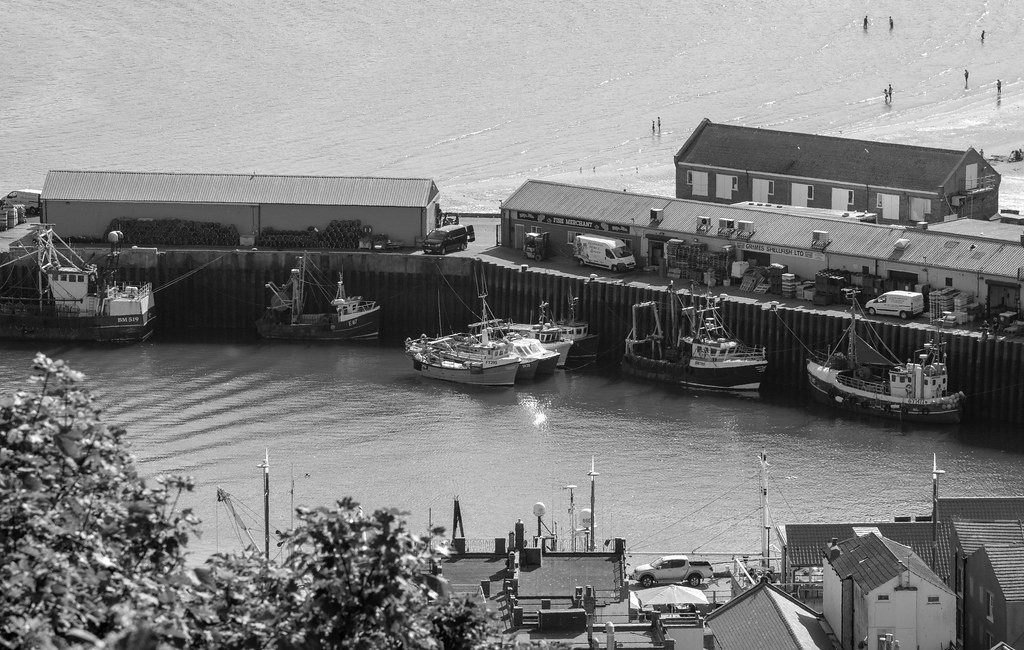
[635,583,710,618]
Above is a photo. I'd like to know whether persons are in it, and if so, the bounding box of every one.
[964,69,970,85]
[979,149,984,158]
[882,83,894,102]
[652,121,656,132]
[657,116,661,132]
[981,30,988,42]
[996,79,1002,94]
[888,16,894,30]
[687,603,695,617]
[746,567,776,585]
[1010,148,1023,161]
[863,15,869,30]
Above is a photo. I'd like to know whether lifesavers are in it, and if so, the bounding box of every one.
[828,388,837,398]
[922,407,929,416]
[623,354,632,364]
[861,401,868,409]
[885,403,891,412]
[850,396,857,405]
[129,295,134,299]
[905,384,912,394]
[902,407,908,414]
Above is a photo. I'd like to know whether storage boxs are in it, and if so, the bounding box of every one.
[658,239,1024,333]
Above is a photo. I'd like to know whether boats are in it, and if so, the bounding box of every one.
[621,279,769,393]
[254,249,381,341]
[404,265,600,386]
[805,289,967,424]
[0,223,158,345]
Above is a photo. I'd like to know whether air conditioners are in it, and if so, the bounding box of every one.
[650,208,663,220]
[719,218,735,229]
[737,220,753,232]
[952,195,967,206]
[697,216,711,226]
[813,230,829,241]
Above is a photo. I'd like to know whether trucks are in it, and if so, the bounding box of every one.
[864,290,924,320]
[0,190,40,215]
[572,233,638,273]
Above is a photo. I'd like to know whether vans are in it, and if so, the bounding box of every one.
[422,224,468,256]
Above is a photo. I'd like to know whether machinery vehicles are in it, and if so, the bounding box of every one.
[523,232,550,262]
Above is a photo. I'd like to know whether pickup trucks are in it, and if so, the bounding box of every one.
[631,555,714,588]
